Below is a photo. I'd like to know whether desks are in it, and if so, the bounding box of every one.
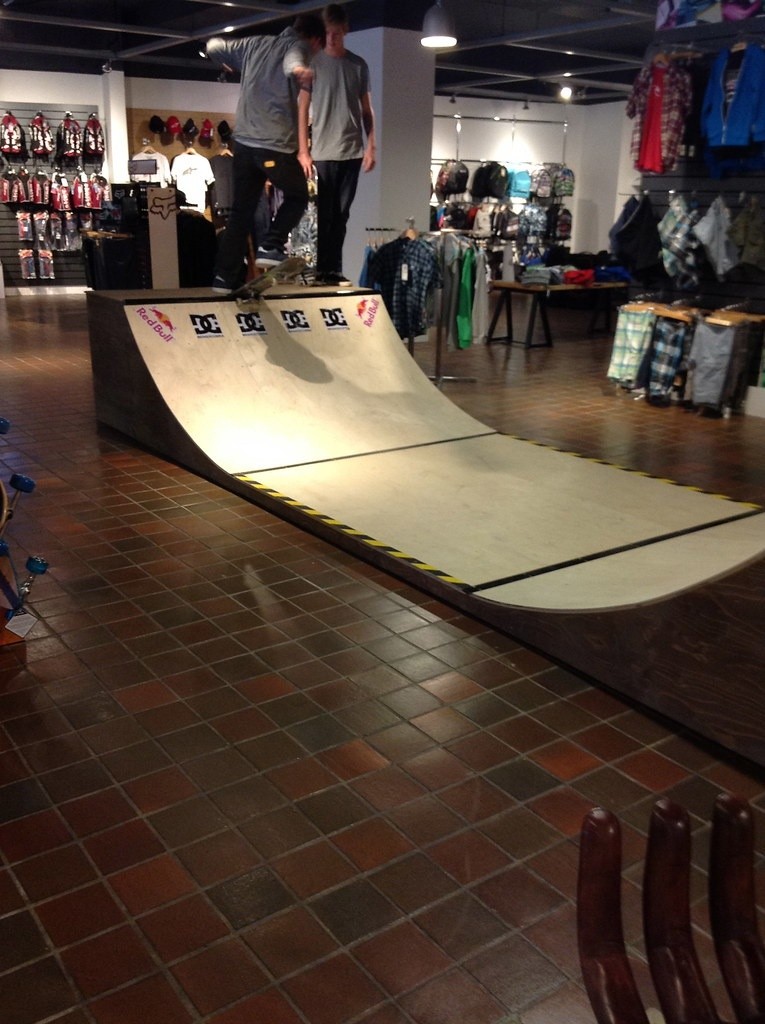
[485,280,625,349]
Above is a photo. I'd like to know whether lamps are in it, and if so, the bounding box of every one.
[420,0,457,49]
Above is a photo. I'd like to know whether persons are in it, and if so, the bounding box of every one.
[206,12,325,293]
[297,4,377,287]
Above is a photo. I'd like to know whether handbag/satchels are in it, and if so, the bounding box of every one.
[517,245,543,266]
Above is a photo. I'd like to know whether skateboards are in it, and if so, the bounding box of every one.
[236,256,307,300]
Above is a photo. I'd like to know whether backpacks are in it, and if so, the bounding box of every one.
[479,163,508,198]
[531,168,551,197]
[551,167,575,196]
[506,168,531,199]
[436,202,572,240]
[436,160,469,193]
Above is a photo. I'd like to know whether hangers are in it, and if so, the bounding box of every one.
[367,225,399,250]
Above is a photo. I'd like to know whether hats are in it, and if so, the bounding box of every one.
[183,118,198,135]
[149,115,165,134]
[166,116,184,134]
[200,118,213,136]
[218,121,231,141]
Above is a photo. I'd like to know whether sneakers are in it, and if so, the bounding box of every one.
[255,247,291,269]
[212,274,259,297]
[314,274,352,287]
